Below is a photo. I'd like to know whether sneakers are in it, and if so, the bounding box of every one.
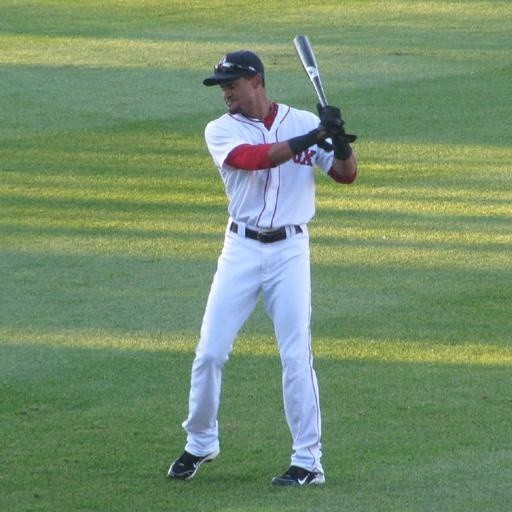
[271,467,326,487]
[167,445,220,481]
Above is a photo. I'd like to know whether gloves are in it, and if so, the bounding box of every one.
[286,104,357,160]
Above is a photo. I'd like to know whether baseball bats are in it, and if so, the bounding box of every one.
[292,34,328,112]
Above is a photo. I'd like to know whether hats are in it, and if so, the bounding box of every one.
[203,51,263,86]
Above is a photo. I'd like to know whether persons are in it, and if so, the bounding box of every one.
[163,48,356,489]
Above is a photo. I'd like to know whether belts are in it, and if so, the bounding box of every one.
[231,222,302,244]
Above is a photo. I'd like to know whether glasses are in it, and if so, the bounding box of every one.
[214,63,257,73]
